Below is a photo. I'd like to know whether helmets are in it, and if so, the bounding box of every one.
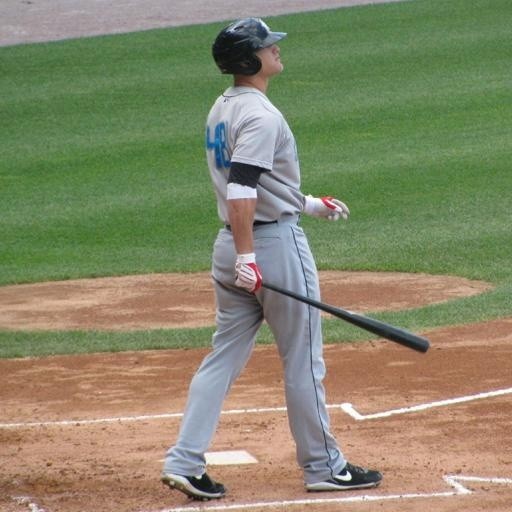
[212,17,289,76]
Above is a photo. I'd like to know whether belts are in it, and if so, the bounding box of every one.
[226,220,278,230]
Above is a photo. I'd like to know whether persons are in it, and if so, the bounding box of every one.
[158,16,384,501]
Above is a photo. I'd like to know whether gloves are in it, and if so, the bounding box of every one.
[234,253,262,293]
[303,194,350,221]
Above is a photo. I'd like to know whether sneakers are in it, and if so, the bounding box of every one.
[304,462,382,491]
[160,469,226,502]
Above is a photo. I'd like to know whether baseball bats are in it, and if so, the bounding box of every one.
[234,273,429,353]
